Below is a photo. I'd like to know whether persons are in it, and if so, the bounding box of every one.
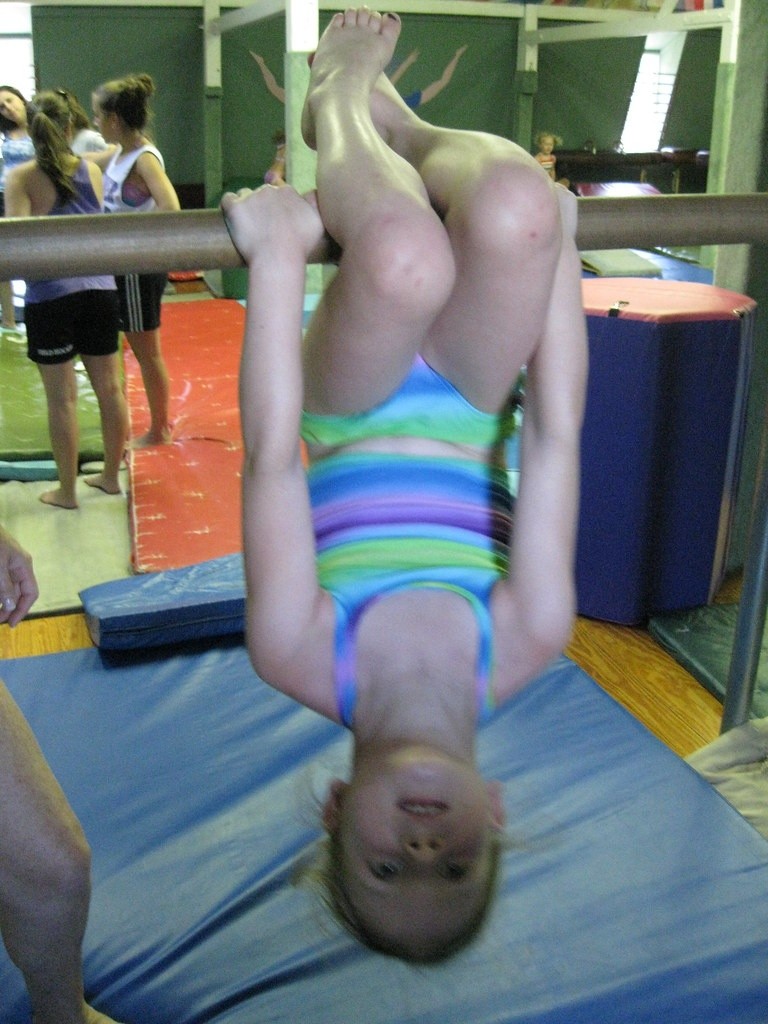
[5,93,126,507]
[222,9,589,953]
[92,76,180,448]
[0,86,37,334]
[535,135,557,181]
[0,527,113,1024]
